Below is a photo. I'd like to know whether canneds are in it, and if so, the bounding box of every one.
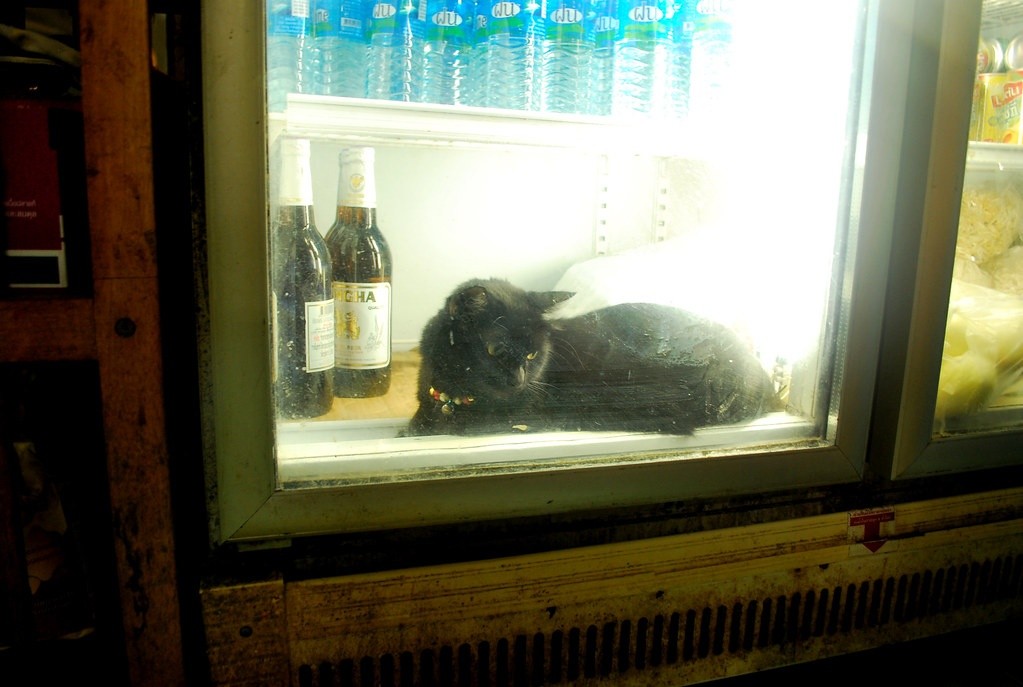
[969,31,1022,146]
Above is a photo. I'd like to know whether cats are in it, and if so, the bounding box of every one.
[398,278,792,437]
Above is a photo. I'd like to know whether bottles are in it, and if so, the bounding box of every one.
[323,145,395,396]
[271,139,334,417]
[265,0,732,125]
[10,439,101,641]
